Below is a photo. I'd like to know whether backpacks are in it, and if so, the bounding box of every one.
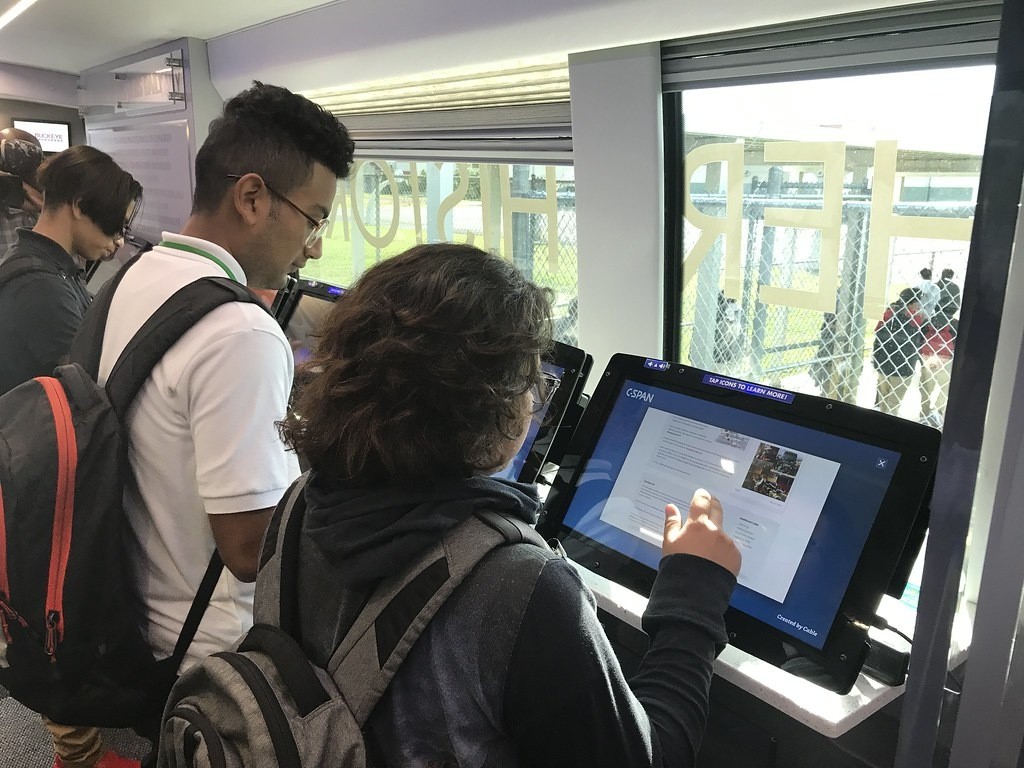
[156,467,567,768]
[0,240,277,729]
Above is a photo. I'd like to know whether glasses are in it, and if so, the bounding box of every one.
[225,175,329,249]
[123,224,131,236]
[520,371,560,404]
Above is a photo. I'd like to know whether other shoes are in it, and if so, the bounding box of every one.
[920,419,929,425]
[927,412,943,430]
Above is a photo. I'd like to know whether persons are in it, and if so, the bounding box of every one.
[0,145,142,768]
[872,269,961,428]
[0,129,45,267]
[65,81,355,768]
[259,243,741,768]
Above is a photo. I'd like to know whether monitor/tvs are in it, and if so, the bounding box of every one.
[247,272,353,373]
[84,234,152,298]
[487,342,945,696]
[11,117,72,156]
